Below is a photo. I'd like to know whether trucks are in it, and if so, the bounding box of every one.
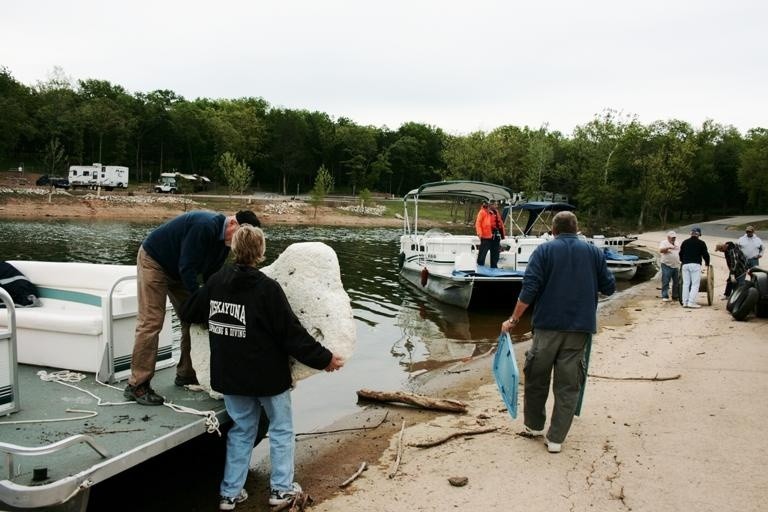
[68,162,130,191]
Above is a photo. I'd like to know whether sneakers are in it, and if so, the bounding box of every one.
[661,296,701,308]
[522,421,544,438]
[122,382,165,406]
[219,488,248,510]
[268,481,303,506]
[545,432,563,454]
[175,374,200,387]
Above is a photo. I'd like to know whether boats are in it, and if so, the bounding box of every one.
[0,258,261,511]
[394,179,645,311]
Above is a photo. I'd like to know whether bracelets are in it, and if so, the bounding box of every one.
[508,315,519,322]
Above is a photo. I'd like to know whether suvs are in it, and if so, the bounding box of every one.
[153,181,179,194]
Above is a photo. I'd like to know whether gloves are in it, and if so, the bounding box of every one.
[730,275,737,283]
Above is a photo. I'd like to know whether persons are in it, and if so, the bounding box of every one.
[473,197,506,270]
[738,224,764,270]
[498,210,615,455]
[123,209,263,406]
[178,223,346,510]
[678,227,710,309]
[714,239,745,299]
[657,230,682,303]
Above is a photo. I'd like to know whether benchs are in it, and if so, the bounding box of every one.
[0,259,172,372]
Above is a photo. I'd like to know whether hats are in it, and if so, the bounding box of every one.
[745,225,754,232]
[237,210,261,232]
[667,230,677,238]
[691,227,701,236]
[715,243,723,252]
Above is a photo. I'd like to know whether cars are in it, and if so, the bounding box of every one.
[35,174,70,189]
